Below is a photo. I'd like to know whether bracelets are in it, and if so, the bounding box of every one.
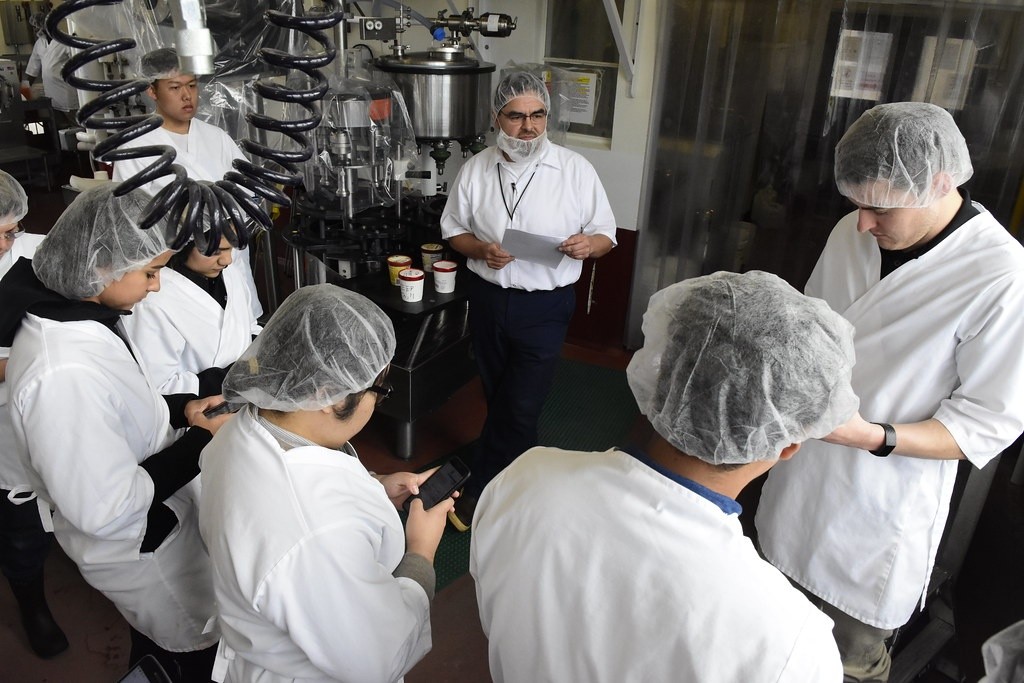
[867,422,897,458]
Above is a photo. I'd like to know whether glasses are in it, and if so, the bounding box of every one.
[499,108,547,126]
[0,221,26,240]
[366,381,395,406]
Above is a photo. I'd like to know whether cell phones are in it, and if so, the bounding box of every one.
[203,401,247,420]
[402,456,471,516]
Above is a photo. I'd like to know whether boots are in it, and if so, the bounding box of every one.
[7,564,70,658]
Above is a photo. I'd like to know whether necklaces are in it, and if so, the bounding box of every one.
[496,163,540,223]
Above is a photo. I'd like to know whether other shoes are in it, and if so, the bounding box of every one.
[447,482,482,531]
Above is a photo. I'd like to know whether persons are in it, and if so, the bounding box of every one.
[21,9,80,129]
[197,276,461,683]
[125,202,263,440]
[467,263,861,683]
[746,94,1024,683]
[111,44,265,339]
[439,70,618,535]
[0,168,221,683]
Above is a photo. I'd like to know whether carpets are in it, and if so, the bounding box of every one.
[398,358,640,594]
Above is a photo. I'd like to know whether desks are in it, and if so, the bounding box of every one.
[331,248,480,460]
